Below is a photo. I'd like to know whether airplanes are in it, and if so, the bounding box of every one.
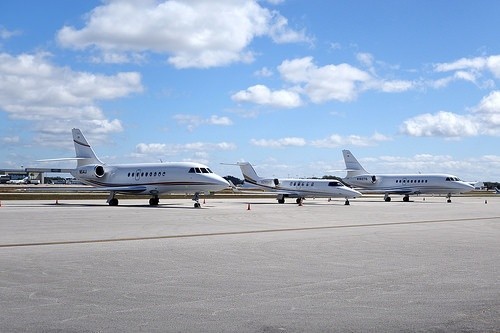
[341,150,475,203]
[229,161,363,205]
[35,128,232,209]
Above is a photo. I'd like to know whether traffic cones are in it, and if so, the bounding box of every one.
[203,199,205,204]
[298,201,302,206]
[247,204,251,210]
